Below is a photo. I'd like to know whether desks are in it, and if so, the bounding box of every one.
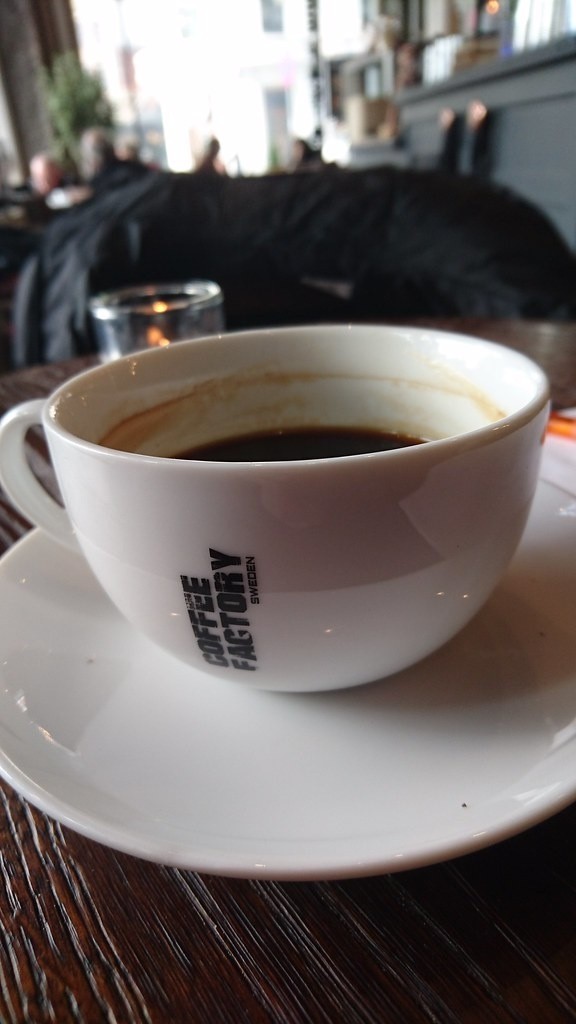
[0,319,576,1024]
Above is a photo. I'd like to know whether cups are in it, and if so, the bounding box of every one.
[87,280,224,365]
[0,324,551,695]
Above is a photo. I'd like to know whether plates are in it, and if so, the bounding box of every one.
[0,431,576,881]
